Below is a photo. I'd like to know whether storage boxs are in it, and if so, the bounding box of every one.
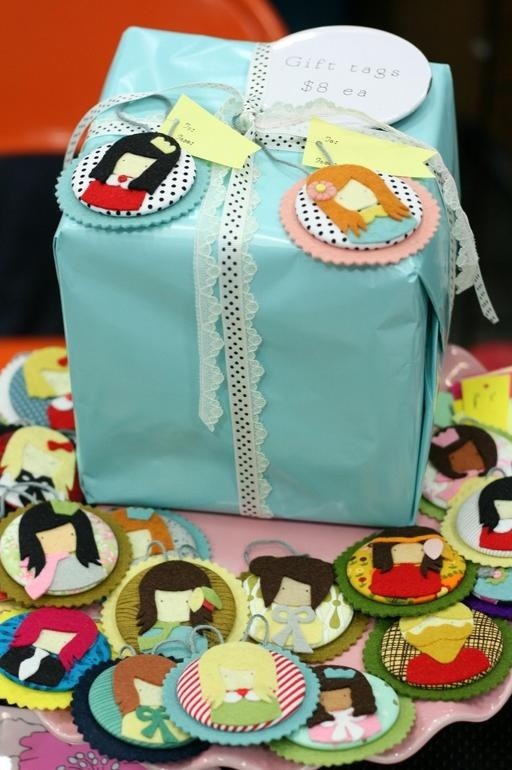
[48,25,500,526]
